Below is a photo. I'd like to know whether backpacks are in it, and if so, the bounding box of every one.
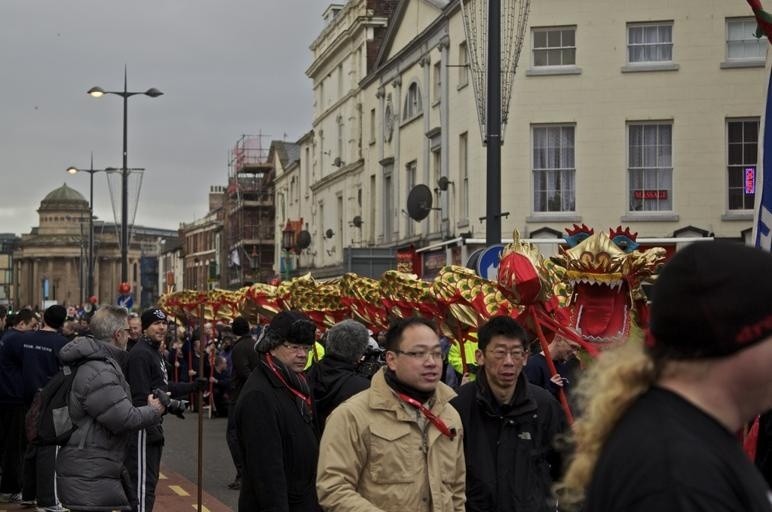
[23,356,114,447]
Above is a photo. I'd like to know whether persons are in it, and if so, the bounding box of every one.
[583,239,772,512]
[225,311,319,512]
[525,329,578,402]
[17,303,70,512]
[304,320,371,439]
[126,316,142,351]
[449,314,563,512]
[228,317,256,491]
[314,315,468,512]
[0,304,8,340]
[0,308,39,502]
[124,308,207,511]
[56,305,171,512]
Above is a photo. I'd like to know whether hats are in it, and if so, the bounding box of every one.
[43,305,67,329]
[141,308,166,334]
[650,240,772,361]
[253,311,317,353]
[232,316,249,336]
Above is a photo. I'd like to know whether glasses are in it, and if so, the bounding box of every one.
[483,346,524,360]
[280,342,311,353]
[123,328,132,334]
[383,346,445,361]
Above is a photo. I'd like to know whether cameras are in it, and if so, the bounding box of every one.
[151,388,190,421]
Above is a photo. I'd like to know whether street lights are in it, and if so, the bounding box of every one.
[83,63,166,309]
[87,62,165,279]
[65,151,115,298]
[65,148,108,303]
